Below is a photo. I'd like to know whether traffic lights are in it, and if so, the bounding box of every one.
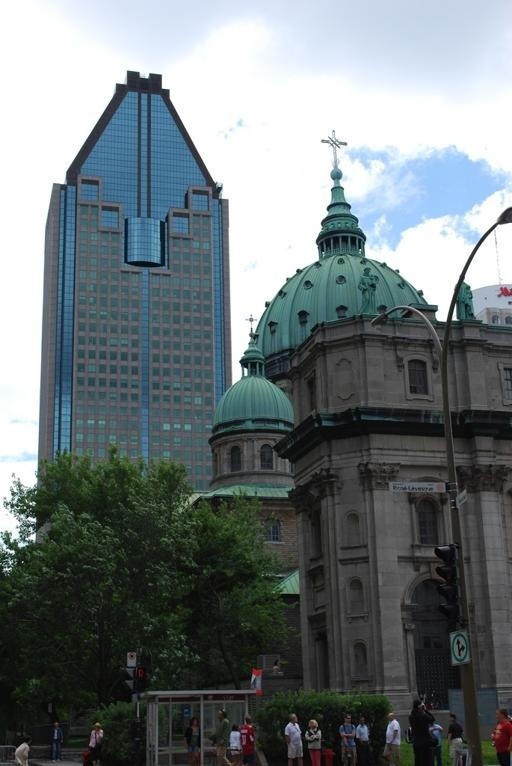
[432,541,459,625]
[135,664,147,693]
[123,668,136,692]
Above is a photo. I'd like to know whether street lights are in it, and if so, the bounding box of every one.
[372,203,510,765]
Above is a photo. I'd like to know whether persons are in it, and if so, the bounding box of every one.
[273,658,285,676]
[210,698,470,765]
[184,716,201,766]
[15,736,31,766]
[88,721,106,765]
[491,707,512,765]
[50,719,64,764]
